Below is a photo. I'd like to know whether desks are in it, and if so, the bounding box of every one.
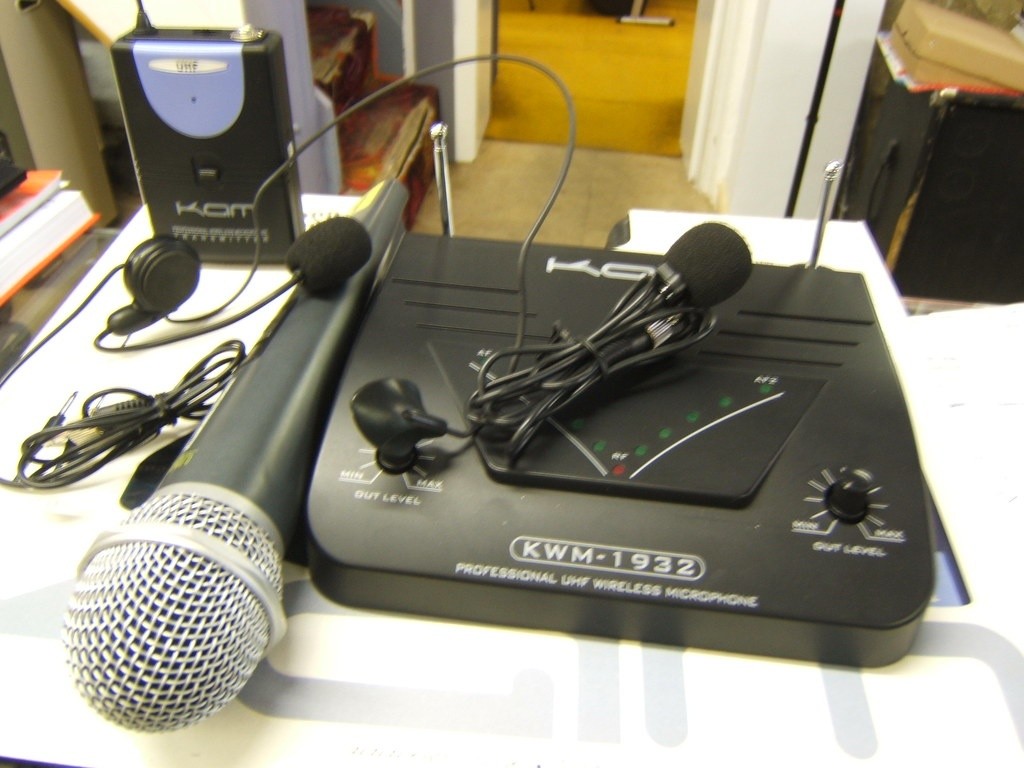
[0,193,1024,768]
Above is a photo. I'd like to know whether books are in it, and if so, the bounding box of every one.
[0,166,101,308]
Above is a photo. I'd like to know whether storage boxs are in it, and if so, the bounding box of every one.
[888,0,1024,93]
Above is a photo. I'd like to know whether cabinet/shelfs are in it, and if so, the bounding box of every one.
[244,0,443,232]
[834,32,1024,302]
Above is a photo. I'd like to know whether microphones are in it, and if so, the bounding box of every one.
[61,179,409,728]
[92,215,372,355]
[655,222,752,307]
